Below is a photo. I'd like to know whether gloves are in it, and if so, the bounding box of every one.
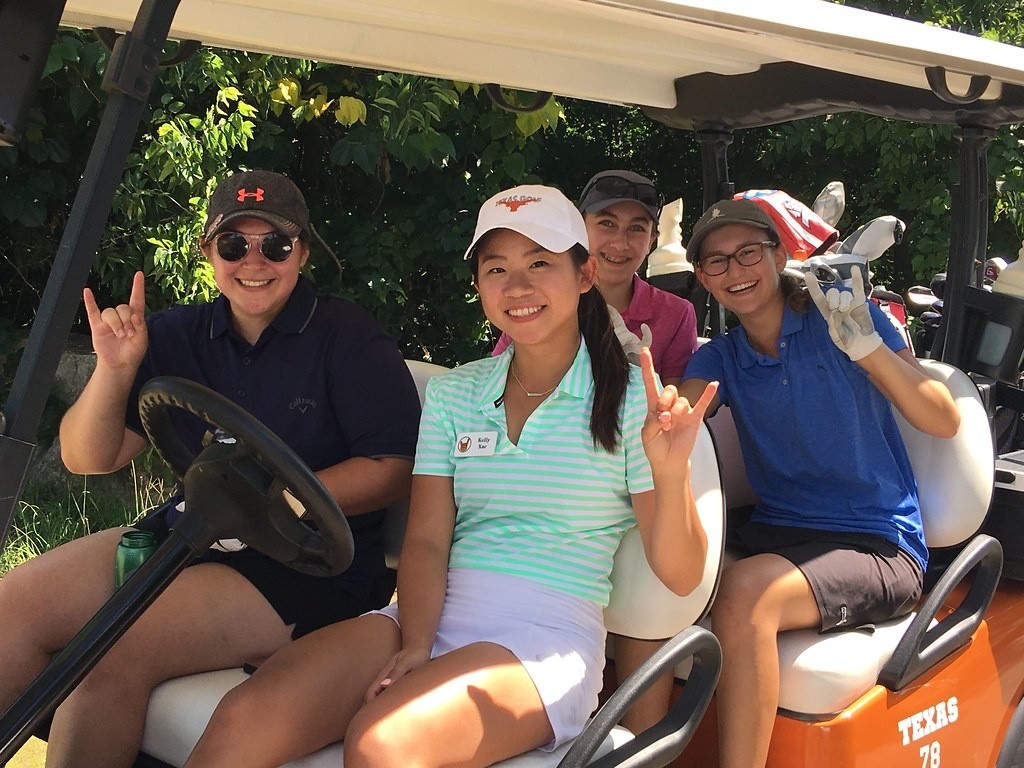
[805,265,884,361]
[175,484,307,552]
[606,304,652,367]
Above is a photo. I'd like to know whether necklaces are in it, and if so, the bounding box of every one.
[511,358,557,397]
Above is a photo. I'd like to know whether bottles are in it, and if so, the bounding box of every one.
[115,531,156,591]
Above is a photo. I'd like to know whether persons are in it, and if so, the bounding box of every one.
[619,199,960,768]
[0,170,423,768]
[488,168,698,385]
[189,185,719,768]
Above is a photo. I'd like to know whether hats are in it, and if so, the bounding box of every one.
[204,170,311,244]
[464,185,589,260]
[686,200,780,263]
[579,170,660,225]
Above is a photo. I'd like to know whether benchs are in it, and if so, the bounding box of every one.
[675,358,1004,719]
[144,361,727,768]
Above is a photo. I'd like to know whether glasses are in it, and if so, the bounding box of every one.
[697,241,776,276]
[215,232,299,264]
[580,176,664,220]
